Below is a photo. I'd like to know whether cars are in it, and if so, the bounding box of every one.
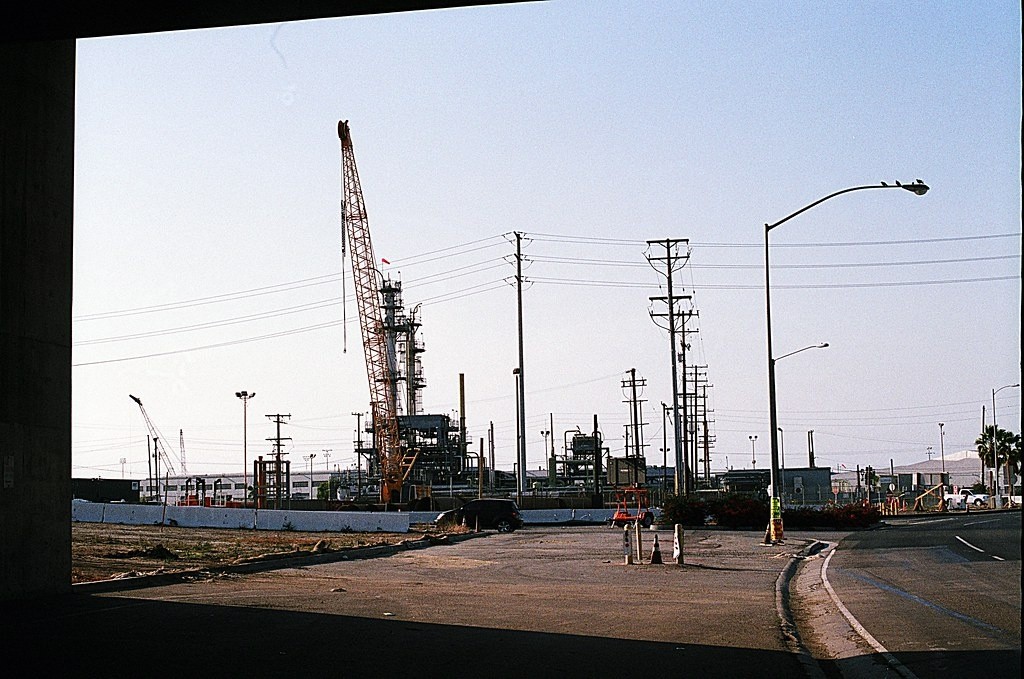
[434,499,524,534]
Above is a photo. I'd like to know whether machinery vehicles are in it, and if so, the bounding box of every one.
[609,485,653,528]
[338,119,432,507]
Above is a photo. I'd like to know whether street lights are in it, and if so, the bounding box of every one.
[749,435,757,469]
[778,427,785,495]
[938,422,944,472]
[925,446,935,467]
[763,178,930,543]
[235,391,256,509]
[772,342,829,413]
[513,369,522,507]
[992,383,1020,495]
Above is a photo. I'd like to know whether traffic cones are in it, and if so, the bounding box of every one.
[651,534,665,565]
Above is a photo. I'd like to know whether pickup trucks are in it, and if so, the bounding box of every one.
[944,489,990,507]
